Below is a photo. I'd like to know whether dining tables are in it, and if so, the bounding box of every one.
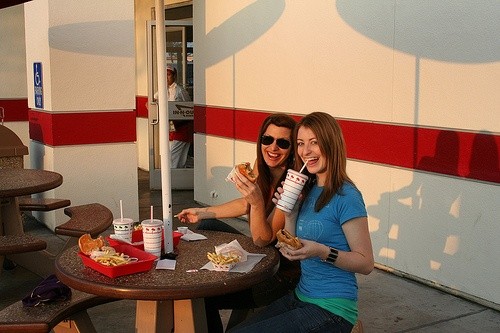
[0,168,64,234]
[54,230,280,333]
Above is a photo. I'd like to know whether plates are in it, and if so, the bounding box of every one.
[107,230,183,250]
[78,239,157,278]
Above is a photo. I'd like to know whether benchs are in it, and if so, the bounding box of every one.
[55,202,113,237]
[18,199,71,211]
[0,287,124,333]
[0,232,47,254]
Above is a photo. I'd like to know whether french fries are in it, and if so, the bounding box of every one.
[206,251,239,264]
[92,249,131,266]
[133,223,142,231]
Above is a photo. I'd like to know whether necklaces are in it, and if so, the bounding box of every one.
[270,184,276,188]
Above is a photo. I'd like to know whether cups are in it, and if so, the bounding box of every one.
[112,218,134,244]
[141,219,162,261]
[275,169,308,214]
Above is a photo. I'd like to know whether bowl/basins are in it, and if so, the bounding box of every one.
[177,227,188,234]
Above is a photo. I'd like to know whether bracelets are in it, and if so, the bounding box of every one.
[321,247,338,265]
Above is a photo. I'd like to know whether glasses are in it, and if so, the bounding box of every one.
[260,136,292,149]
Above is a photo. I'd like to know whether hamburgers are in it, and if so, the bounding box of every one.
[234,162,257,184]
[78,233,106,256]
[276,229,302,250]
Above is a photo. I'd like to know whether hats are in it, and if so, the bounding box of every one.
[167,62,177,71]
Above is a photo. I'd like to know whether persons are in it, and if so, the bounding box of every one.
[145,65,194,168]
[178,114,301,333]
[225,112,374,333]
